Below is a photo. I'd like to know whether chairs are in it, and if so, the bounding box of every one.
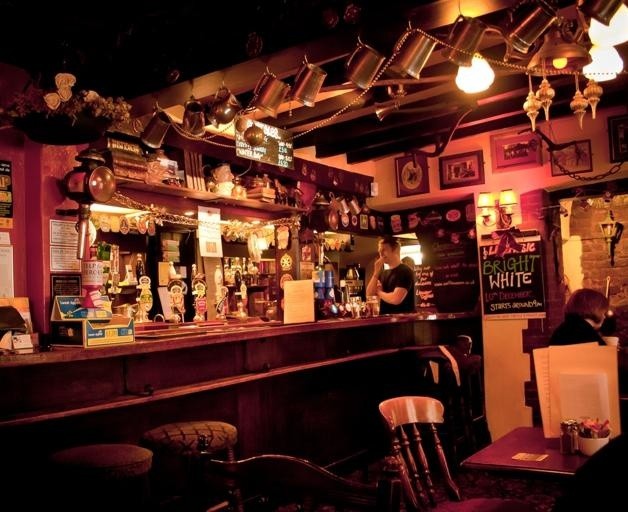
[377,396,529,512]
[236,454,402,512]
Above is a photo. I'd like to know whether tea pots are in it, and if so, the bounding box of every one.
[346,264,362,281]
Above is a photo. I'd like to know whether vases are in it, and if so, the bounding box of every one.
[9,118,114,146]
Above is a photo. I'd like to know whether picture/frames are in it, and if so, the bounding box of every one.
[490,125,544,174]
[393,154,430,198]
[437,149,486,190]
[550,138,593,177]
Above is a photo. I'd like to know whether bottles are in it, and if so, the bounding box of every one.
[241,257,247,274]
[234,269,240,286]
[168,261,176,280]
[246,257,253,274]
[190,263,197,281]
[135,253,145,280]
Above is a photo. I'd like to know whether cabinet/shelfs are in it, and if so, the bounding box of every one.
[88,122,375,322]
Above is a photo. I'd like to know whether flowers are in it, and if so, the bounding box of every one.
[0,73,133,132]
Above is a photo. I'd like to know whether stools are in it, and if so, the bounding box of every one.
[56,444,154,481]
[137,420,238,512]
[418,354,484,433]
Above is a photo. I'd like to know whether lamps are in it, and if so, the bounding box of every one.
[477,189,519,227]
[523,22,602,132]
[347,192,364,215]
[501,0,558,54]
[231,177,245,200]
[87,166,116,203]
[253,65,291,119]
[345,36,387,90]
[441,0,488,67]
[182,94,206,137]
[289,53,328,108]
[454,50,495,94]
[336,191,353,214]
[606,113,627,164]
[597,210,622,264]
[140,101,171,149]
[577,0,624,26]
[588,2,628,47]
[207,80,243,129]
[392,20,439,80]
[373,84,407,121]
[583,46,625,83]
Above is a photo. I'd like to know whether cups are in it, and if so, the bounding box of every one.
[601,337,619,351]
[351,305,361,320]
[350,295,359,304]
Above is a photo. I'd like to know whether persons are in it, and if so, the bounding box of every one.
[366,238,416,316]
[548,289,613,348]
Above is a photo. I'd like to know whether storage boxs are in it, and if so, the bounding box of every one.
[49,317,135,348]
